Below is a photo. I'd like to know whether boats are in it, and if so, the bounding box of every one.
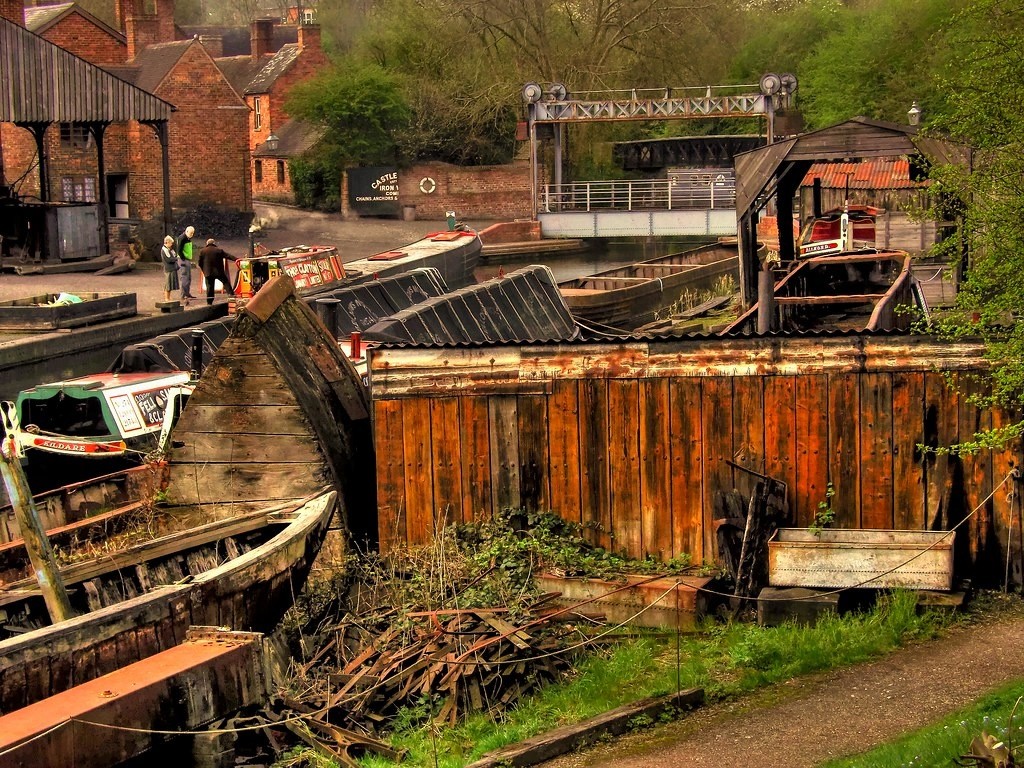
[0,207,917,718]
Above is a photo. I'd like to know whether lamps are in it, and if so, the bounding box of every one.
[242,128,279,162]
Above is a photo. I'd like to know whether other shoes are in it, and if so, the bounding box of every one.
[183,296,197,300]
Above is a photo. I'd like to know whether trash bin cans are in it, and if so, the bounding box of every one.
[401,204,417,221]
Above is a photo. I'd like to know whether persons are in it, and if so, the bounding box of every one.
[161,235,181,302]
[176,225,197,300]
[198,238,239,307]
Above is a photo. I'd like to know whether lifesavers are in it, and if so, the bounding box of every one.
[420,177,435,194]
